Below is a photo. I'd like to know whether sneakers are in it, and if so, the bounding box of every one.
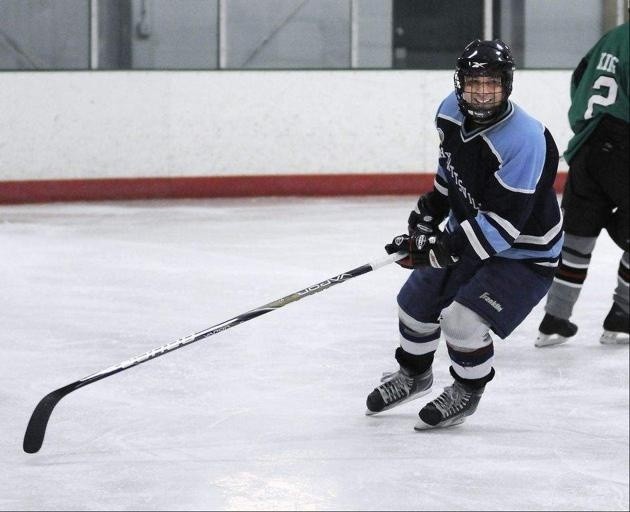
[602,303,630,334]
[538,312,580,338]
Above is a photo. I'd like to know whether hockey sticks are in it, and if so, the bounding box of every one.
[22,248,410,453]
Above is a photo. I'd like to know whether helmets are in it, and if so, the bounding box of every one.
[452,36,516,126]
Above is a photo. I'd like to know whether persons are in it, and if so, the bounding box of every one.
[536,19,630,336]
[365,38,567,428]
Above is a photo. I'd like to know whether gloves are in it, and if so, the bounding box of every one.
[383,226,462,271]
[405,188,450,236]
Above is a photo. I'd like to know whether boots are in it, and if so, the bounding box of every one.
[362,345,435,412]
[417,367,496,426]
